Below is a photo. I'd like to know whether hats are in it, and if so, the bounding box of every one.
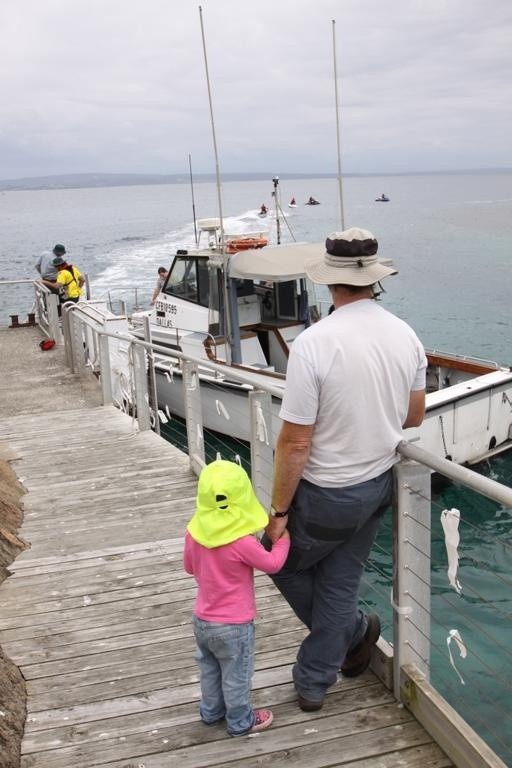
[185,460,269,550]
[51,257,64,265]
[53,244,66,256]
[304,228,398,287]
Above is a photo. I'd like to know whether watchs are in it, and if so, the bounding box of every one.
[270,505,288,517]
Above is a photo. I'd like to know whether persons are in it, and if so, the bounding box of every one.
[260,228,428,711]
[183,460,290,737]
[150,267,176,305]
[35,244,85,316]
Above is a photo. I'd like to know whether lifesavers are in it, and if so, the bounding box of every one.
[227,235,267,250]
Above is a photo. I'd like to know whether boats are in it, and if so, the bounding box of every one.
[287,200,321,208]
[375,198,390,201]
[97,217,512,478]
[258,211,267,218]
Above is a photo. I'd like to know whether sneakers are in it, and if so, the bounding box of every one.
[342,613,381,678]
[298,695,321,710]
[229,709,274,736]
